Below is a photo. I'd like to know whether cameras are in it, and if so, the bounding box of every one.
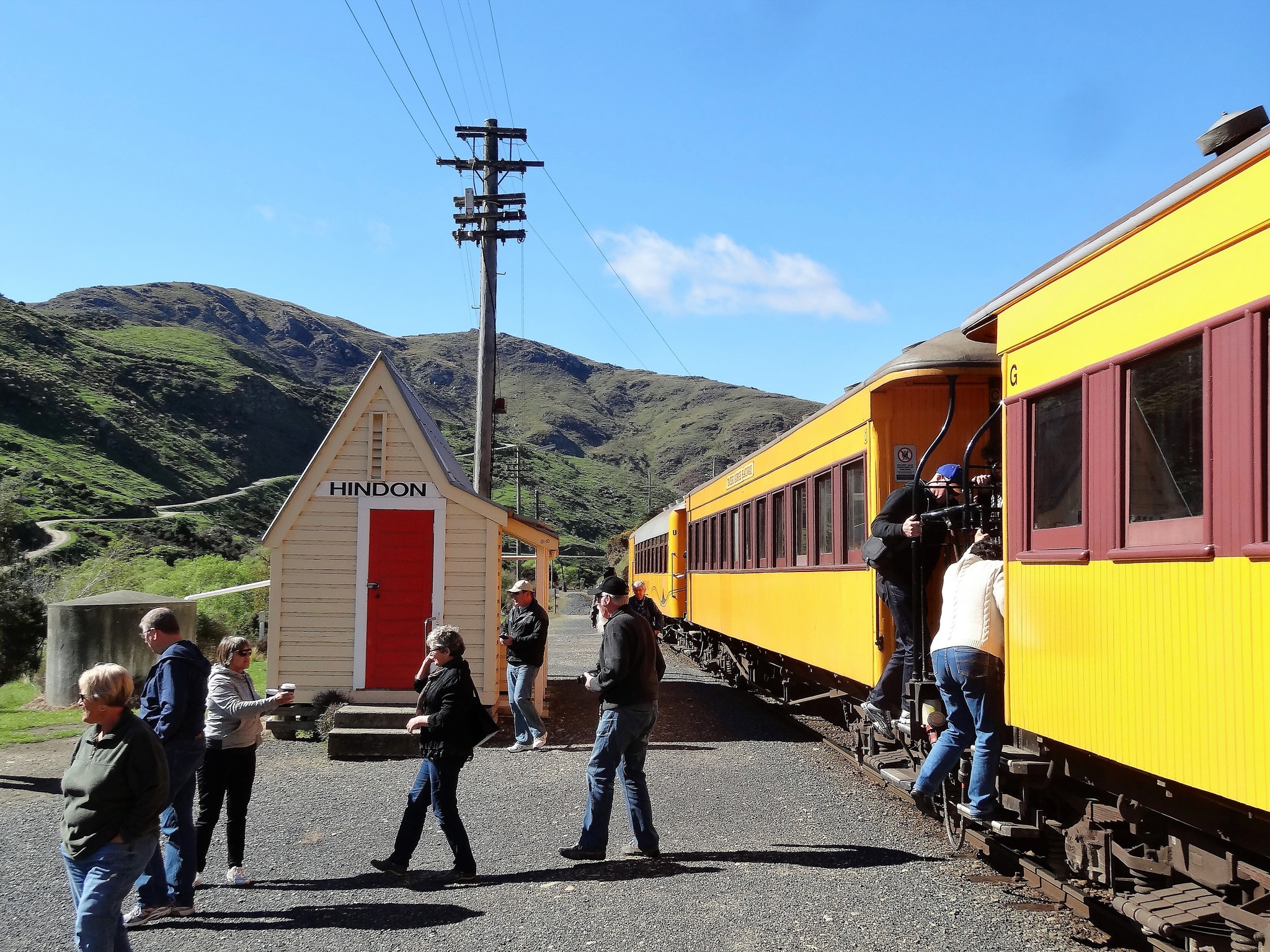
[578,669,600,687]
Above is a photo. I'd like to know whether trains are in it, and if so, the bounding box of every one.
[624,119,1270,952]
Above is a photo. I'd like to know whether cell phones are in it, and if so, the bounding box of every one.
[498,634,509,640]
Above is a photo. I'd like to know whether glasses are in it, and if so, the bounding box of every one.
[233,648,252,657]
[943,476,960,497]
[138,629,160,636]
[430,647,451,654]
[595,595,602,600]
[80,693,100,700]
[513,591,523,595]
[633,589,644,592]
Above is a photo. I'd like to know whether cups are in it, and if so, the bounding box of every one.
[266,688,279,699]
[281,683,296,695]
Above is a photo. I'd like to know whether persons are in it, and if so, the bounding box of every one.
[590,567,663,638]
[124,608,210,926]
[372,625,476,882]
[557,575,666,858]
[194,637,294,888]
[500,580,549,753]
[58,662,170,952]
[861,465,990,738]
[911,529,1020,820]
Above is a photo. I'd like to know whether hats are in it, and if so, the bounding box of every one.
[506,580,534,594]
[587,575,629,595]
[937,464,963,493]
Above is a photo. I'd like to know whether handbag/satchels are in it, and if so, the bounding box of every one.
[861,534,893,570]
[429,697,500,764]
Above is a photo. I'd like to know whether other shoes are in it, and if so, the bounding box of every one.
[911,788,934,814]
[971,803,1020,821]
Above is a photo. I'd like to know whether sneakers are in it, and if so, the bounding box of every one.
[371,856,406,875]
[532,731,547,749]
[899,710,911,724]
[861,701,895,740]
[620,843,660,855]
[169,902,194,916]
[507,743,533,752]
[558,844,606,861]
[437,868,476,882]
[227,866,250,884]
[123,906,171,926]
[192,872,202,887]
